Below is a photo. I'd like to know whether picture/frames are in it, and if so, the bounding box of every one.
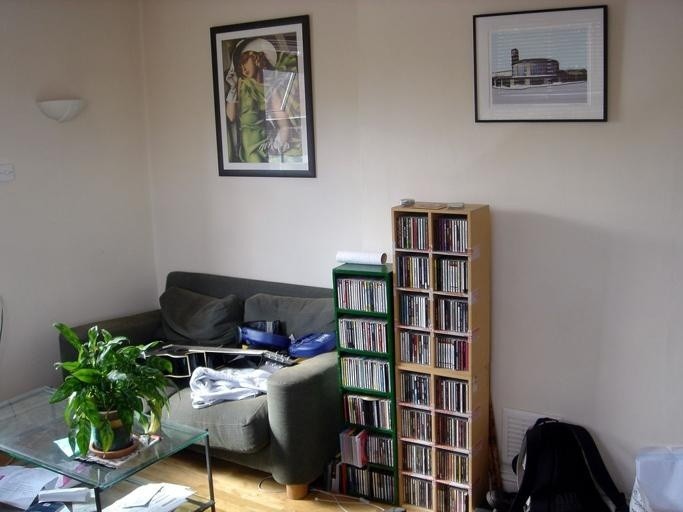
[469,3,609,123]
[208,13,316,179]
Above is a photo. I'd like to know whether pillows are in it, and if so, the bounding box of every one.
[243,292,337,341]
[150,286,242,349]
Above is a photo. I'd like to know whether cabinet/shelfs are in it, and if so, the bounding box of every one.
[331,263,399,508]
[389,201,493,512]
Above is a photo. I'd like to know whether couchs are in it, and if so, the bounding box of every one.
[57,270,346,502]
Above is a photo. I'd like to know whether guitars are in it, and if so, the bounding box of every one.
[135,339,299,378]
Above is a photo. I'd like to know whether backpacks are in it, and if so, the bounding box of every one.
[516,418,628,512]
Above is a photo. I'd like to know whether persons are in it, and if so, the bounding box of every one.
[222,50,291,164]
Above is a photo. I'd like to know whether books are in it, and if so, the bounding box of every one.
[0,462,196,512]
[390,213,469,511]
[325,275,395,502]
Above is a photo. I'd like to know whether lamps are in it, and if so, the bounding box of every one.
[35,96,87,124]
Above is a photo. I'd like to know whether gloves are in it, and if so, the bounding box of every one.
[225,71,237,103]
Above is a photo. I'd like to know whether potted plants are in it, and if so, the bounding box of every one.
[46,319,175,460]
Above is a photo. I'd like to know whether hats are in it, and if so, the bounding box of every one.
[230,38,276,74]
